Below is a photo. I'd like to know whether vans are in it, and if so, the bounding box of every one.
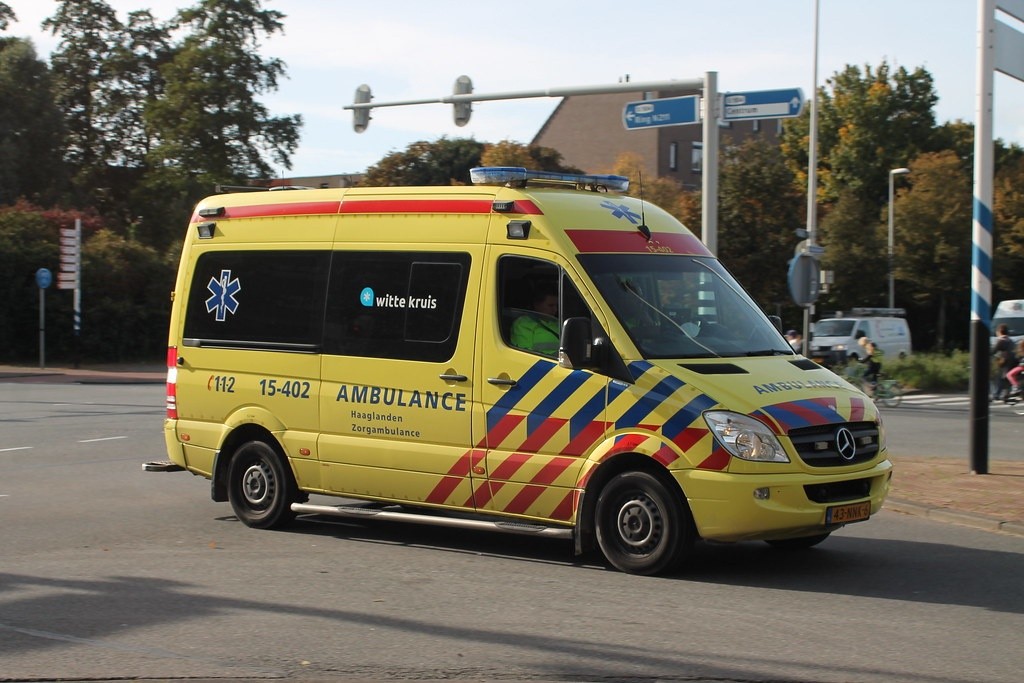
[149,170,894,576]
[989,299,1024,355]
[809,306,912,364]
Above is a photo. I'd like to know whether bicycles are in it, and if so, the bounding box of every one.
[989,356,1024,418]
[840,372,902,409]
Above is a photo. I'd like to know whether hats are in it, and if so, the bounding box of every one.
[788,330,796,335]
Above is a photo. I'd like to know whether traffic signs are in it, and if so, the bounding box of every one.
[724,88,805,121]
[623,95,702,132]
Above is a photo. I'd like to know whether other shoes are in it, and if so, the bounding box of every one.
[1010,388,1020,394]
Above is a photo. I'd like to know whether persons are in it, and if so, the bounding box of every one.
[510,285,560,358]
[787,329,802,353]
[1007,339,1024,395]
[989,324,1017,399]
[615,280,685,353]
[855,329,882,400]
